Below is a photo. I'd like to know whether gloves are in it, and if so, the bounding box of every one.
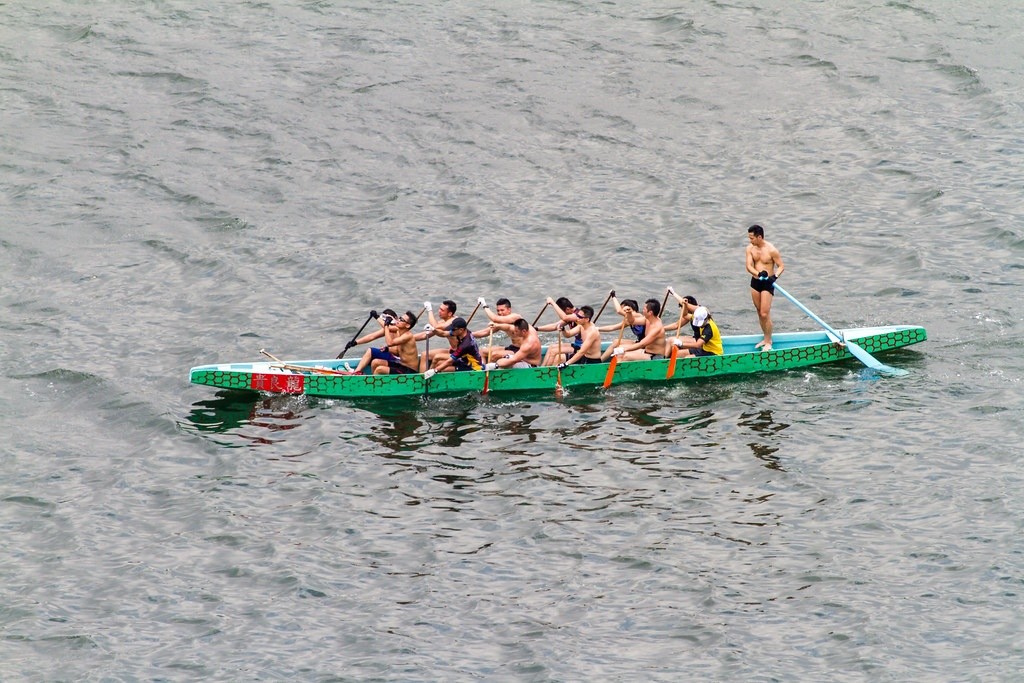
[767,274,778,284]
[610,290,616,298]
[424,301,432,311]
[345,340,357,349]
[380,347,389,353]
[556,361,569,371]
[384,317,392,325]
[673,338,682,347]
[424,324,434,331]
[370,310,380,320]
[611,347,625,356]
[424,369,436,379]
[758,270,768,277]
[478,297,489,309]
[486,363,496,369]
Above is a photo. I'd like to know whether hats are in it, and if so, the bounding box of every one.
[447,318,467,329]
[692,307,707,326]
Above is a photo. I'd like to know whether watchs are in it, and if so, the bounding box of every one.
[483,305,489,309]
[495,363,499,369]
[774,273,778,278]
[434,368,438,373]
[565,362,568,366]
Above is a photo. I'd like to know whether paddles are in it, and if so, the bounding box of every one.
[422,326,435,400]
[602,309,628,388]
[658,291,671,318]
[418,305,427,321]
[760,273,909,379]
[336,314,373,359]
[466,302,482,327]
[480,325,494,398]
[259,346,307,376]
[530,299,552,328]
[554,327,565,401]
[592,289,616,325]
[665,298,688,380]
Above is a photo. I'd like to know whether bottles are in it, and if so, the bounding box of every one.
[381,314,397,323]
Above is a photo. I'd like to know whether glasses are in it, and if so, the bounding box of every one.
[452,328,459,331]
[399,317,410,324]
[576,313,589,319]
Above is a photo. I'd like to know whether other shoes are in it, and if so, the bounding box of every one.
[344,362,354,372]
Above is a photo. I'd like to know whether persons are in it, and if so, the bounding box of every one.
[344,286,723,375]
[746,225,785,351]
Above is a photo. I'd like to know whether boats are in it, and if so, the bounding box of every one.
[189,325,926,397]
